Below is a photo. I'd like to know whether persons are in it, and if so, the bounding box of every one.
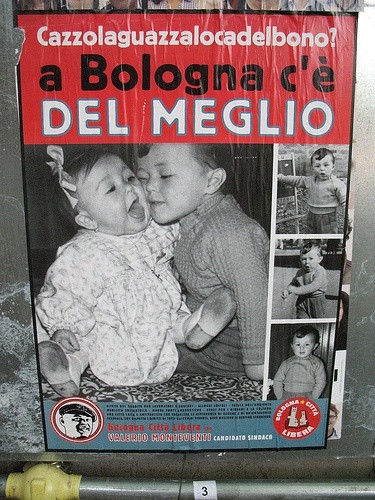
[279,241,331,320]
[36,144,240,400]
[278,146,349,234]
[272,320,330,401]
[327,403,339,440]
[129,139,267,383]
